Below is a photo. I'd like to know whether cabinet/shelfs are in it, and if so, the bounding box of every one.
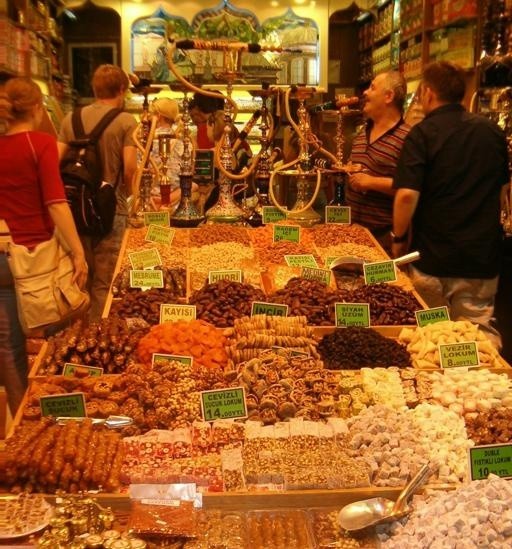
[396,0,476,83]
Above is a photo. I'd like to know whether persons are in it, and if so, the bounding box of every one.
[388,57,511,348]
[340,67,414,259]
[1,76,92,342]
[142,84,293,218]
[0,246,30,419]
[54,63,141,316]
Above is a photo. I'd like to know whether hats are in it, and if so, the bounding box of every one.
[152,96,179,122]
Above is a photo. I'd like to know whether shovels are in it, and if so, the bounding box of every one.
[329,251,421,273]
[339,464,430,532]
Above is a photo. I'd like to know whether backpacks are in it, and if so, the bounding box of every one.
[58,105,124,237]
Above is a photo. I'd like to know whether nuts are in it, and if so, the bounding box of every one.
[0,265,512,549]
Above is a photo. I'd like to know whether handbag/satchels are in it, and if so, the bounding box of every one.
[7,225,91,339]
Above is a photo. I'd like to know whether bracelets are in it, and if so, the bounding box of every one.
[390,231,409,243]
